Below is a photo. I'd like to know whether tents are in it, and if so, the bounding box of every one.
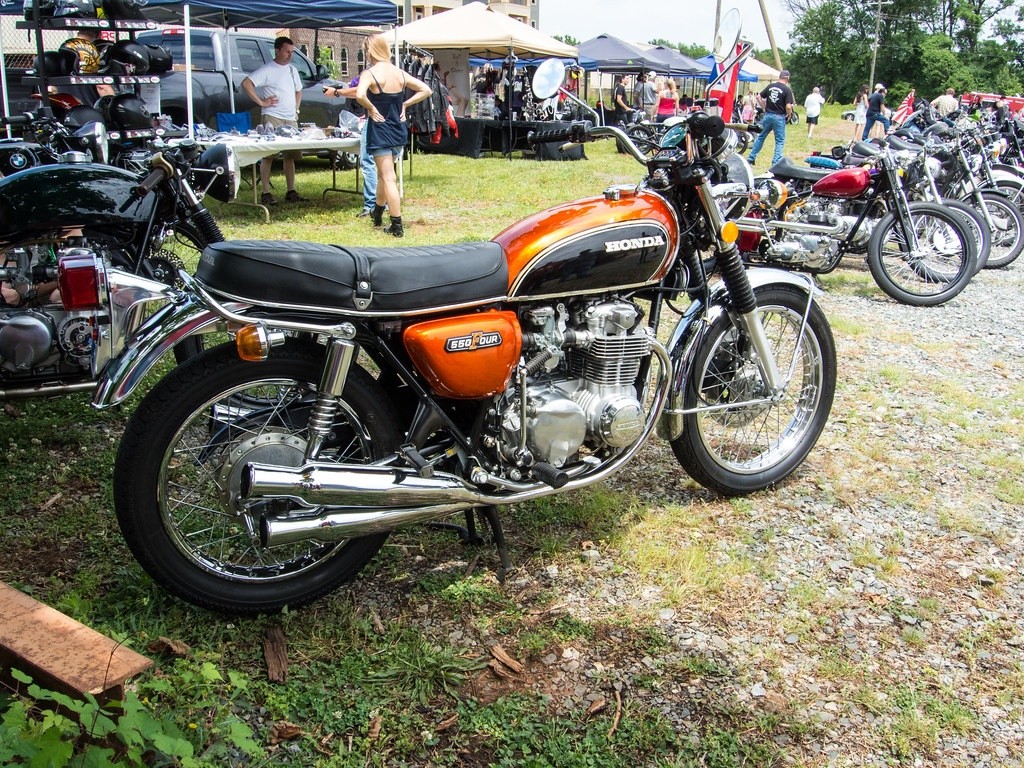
[371,2,578,160]
[573,34,759,150]
[0,0,403,223]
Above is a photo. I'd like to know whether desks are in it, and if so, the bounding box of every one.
[168,136,365,224]
[416,117,589,162]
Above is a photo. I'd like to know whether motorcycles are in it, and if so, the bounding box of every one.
[716,97,1024,306]
[0,86,362,410]
[89,7,842,618]
[616,112,758,160]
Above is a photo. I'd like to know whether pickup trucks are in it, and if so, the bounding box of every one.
[22,28,359,160]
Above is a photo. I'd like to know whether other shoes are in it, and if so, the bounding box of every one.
[357,209,370,218]
[747,159,755,165]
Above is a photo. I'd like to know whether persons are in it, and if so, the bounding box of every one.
[969,94,982,115]
[732,89,759,128]
[747,70,794,167]
[804,87,825,138]
[993,95,1009,124]
[612,69,686,155]
[241,37,309,205]
[930,88,959,118]
[323,33,432,237]
[853,82,895,143]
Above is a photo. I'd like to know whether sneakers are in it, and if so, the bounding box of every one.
[285,191,310,202]
[261,192,279,205]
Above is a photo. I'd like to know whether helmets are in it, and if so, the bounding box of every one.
[22,1,175,133]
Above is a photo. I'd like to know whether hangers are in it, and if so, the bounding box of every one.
[504,47,518,63]
[405,43,434,66]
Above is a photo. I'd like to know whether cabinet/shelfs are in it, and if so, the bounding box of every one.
[16,0,198,141]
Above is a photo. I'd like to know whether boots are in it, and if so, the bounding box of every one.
[384,216,404,238]
[370,203,386,227]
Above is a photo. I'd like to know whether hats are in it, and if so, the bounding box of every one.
[878,88,888,96]
[778,69,790,78]
[648,71,657,79]
[874,84,884,88]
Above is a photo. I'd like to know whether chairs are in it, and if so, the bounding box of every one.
[217,112,276,192]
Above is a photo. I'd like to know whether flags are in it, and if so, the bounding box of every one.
[891,90,915,125]
